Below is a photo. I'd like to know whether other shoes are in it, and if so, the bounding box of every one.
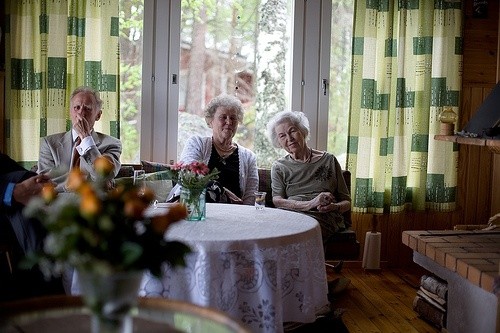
[284,322,305,331]
[315,305,328,318]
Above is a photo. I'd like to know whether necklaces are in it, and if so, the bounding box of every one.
[304,148,312,163]
[212,137,234,156]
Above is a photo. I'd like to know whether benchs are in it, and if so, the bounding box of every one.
[29,161,361,273]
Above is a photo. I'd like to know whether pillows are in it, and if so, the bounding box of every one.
[113,170,172,203]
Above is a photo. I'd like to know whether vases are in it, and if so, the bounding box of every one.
[67,253,142,333]
[180,187,207,221]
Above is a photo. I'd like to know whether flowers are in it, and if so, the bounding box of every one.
[19,154,194,282]
[167,161,222,219]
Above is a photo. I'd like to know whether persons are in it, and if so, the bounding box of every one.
[36,86,122,191]
[0,152,65,296]
[266,111,351,261]
[177,94,260,206]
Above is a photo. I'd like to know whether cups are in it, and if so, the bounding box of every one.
[440,123,455,135]
[254,192,267,211]
[147,199,158,209]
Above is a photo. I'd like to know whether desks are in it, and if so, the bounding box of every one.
[0,294,251,333]
[71,203,329,333]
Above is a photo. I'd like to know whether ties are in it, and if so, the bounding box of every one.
[71,136,81,171]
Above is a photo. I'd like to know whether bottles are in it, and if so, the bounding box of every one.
[438,106,457,123]
[133,170,146,196]
